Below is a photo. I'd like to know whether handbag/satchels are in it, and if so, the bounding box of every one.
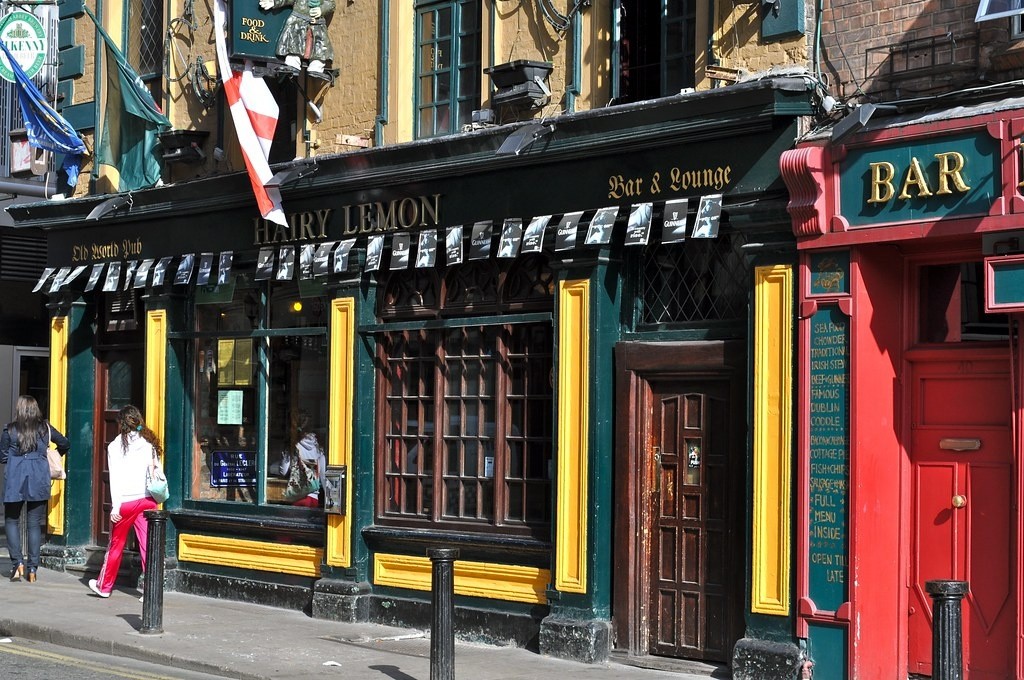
[45,422,67,480]
[282,454,321,502]
[146,448,170,503]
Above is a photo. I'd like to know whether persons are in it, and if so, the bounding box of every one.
[0,394,70,583]
[280,406,325,508]
[89,404,169,602]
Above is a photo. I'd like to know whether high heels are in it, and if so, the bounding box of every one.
[26,567,37,583]
[10,561,25,580]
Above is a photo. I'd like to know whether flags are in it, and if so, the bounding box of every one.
[81,3,173,193]
[212,0,290,229]
[0,38,90,188]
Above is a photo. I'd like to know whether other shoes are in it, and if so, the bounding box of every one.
[89,579,111,598]
[139,595,143,603]
